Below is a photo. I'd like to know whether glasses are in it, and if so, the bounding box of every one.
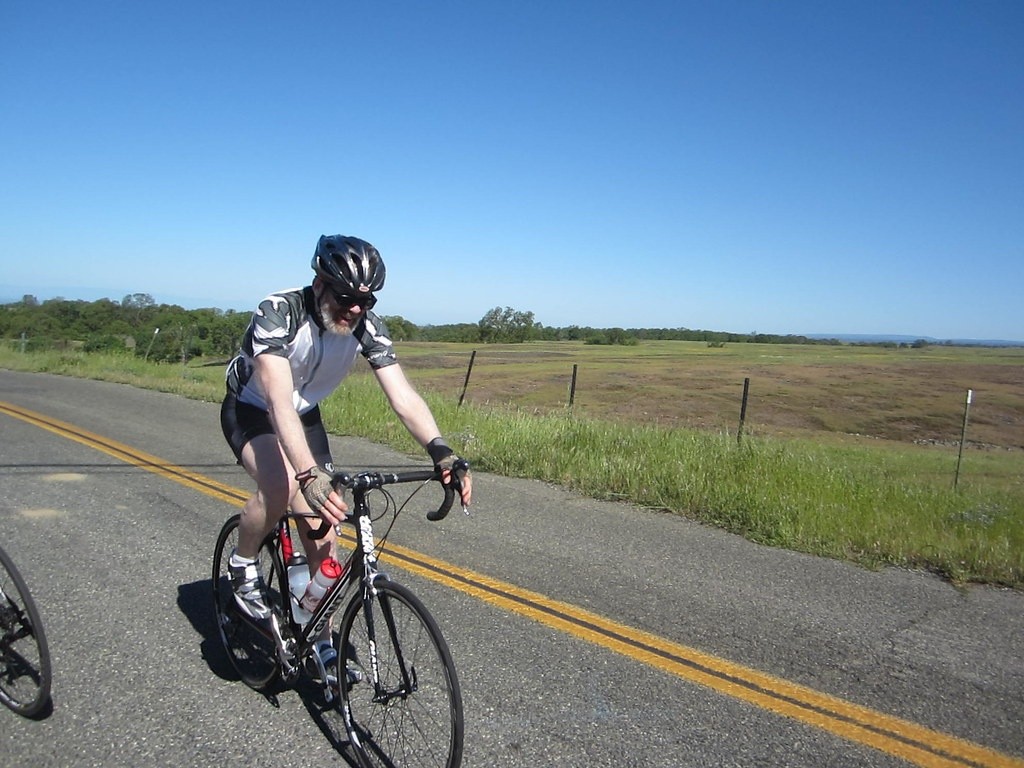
[324,281,377,310]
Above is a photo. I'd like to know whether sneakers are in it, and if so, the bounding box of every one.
[228,550,272,620]
[306,644,362,684]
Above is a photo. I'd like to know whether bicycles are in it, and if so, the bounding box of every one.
[1,548,53,717]
[210,460,471,768]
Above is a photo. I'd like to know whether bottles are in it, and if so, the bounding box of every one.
[302,557,343,612]
[287,552,313,623]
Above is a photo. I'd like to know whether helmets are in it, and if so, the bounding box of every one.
[310,235,386,292]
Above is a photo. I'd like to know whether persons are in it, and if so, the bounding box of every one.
[220,235,471,685]
